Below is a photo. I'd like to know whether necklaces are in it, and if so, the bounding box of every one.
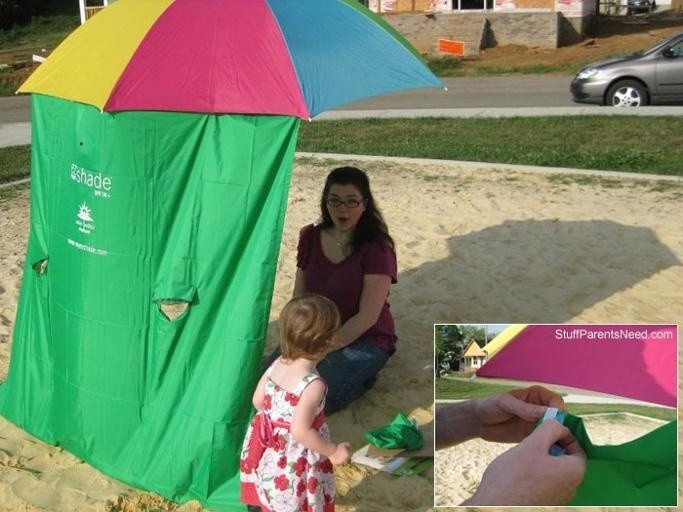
[329,226,353,247]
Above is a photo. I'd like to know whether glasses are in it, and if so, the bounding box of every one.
[325,198,364,208]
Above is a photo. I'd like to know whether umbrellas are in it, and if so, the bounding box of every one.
[14,0,450,122]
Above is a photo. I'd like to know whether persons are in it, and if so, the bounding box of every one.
[262,166,398,414]
[435,386,589,506]
[240,294,353,512]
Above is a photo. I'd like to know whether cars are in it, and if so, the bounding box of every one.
[568,29,683,108]
[627,0,657,16]
